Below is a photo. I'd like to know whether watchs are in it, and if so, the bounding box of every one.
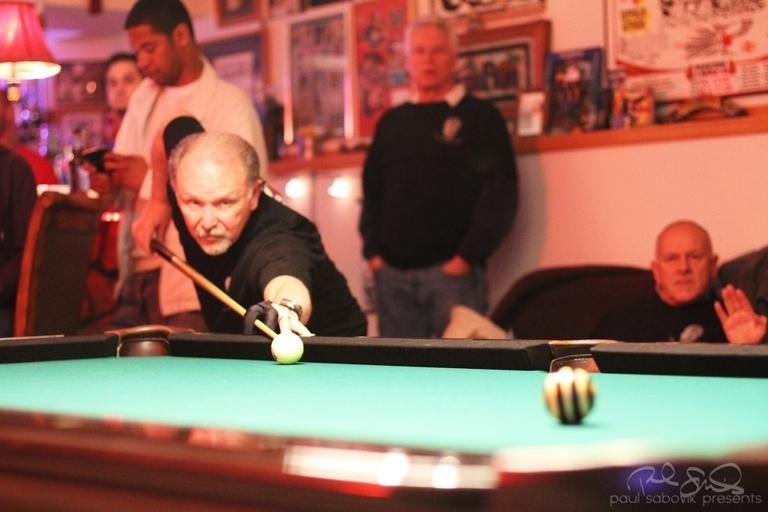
[276,298,303,322]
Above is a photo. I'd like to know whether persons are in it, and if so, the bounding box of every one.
[127,111,368,339]
[591,220,768,344]
[100,51,144,151]
[358,15,519,338]
[77,0,269,332]
[0,91,37,338]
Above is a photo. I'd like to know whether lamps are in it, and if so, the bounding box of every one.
[0,0,63,81]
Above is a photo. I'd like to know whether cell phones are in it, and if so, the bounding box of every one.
[81,146,110,171]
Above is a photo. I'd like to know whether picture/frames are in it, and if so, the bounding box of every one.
[44,55,108,159]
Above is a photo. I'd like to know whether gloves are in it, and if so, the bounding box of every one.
[242,297,315,338]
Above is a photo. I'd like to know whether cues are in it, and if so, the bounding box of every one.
[149,239,278,341]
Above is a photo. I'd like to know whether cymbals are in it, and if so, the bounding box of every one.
[271,332,305,361]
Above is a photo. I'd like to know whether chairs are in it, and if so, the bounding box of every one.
[491,245,768,346]
[12,193,104,337]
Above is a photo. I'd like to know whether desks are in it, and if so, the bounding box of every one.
[0,324,768,512]
